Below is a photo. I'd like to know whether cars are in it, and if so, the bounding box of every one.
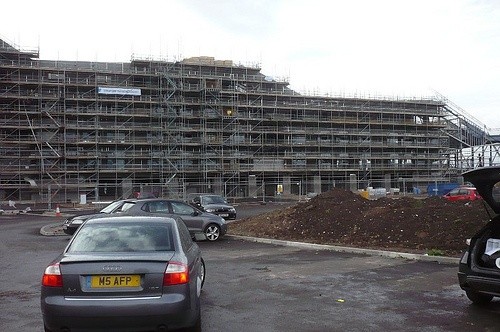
[40,214,206,332]
[62,199,124,235]
[457,166,500,304]
[123,199,228,242]
[440,186,484,201]
[189,194,237,220]
